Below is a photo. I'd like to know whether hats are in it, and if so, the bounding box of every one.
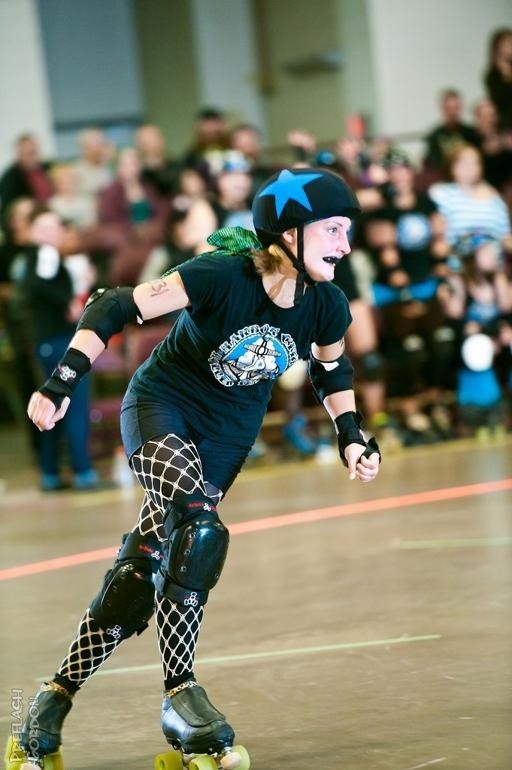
[220,149,256,174]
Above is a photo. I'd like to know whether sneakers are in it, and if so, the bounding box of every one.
[315,436,336,466]
[42,474,65,490]
[71,467,100,490]
[110,446,135,489]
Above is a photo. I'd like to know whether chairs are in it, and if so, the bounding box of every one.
[63,213,182,463]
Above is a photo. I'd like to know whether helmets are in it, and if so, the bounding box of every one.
[251,167,362,248]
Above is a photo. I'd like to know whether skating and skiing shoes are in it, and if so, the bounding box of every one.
[3,680,74,770]
[151,686,251,769]
[364,404,508,452]
[243,413,317,469]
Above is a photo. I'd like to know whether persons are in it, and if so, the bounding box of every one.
[480,26,512,136]
[4,166,380,759]
